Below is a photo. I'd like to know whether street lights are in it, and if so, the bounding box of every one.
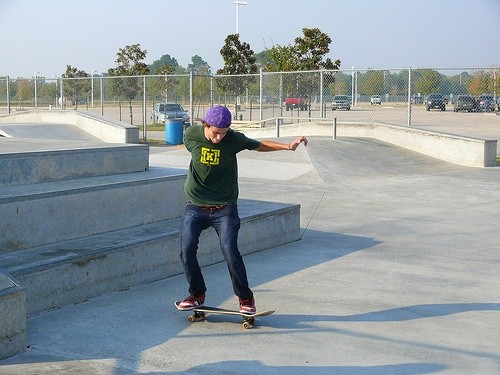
[234,2,247,106]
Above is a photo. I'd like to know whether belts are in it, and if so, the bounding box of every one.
[201,206,221,211]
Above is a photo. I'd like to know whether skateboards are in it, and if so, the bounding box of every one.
[174,300,276,328]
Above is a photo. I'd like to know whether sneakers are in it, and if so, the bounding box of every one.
[239,296,256,314]
[178,294,206,310]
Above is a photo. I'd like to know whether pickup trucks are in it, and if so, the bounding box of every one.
[285,92,310,110]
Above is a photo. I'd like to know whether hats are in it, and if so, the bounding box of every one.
[201,106,231,128]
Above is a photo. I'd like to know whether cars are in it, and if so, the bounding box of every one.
[413,96,424,105]
[425,94,445,111]
[369,95,382,106]
[150,102,189,124]
[330,94,351,111]
[444,98,448,104]
[452,95,500,113]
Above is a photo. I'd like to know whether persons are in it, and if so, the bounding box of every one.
[177,106,307,313]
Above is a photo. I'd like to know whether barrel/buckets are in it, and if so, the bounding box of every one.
[165,118,184,145]
